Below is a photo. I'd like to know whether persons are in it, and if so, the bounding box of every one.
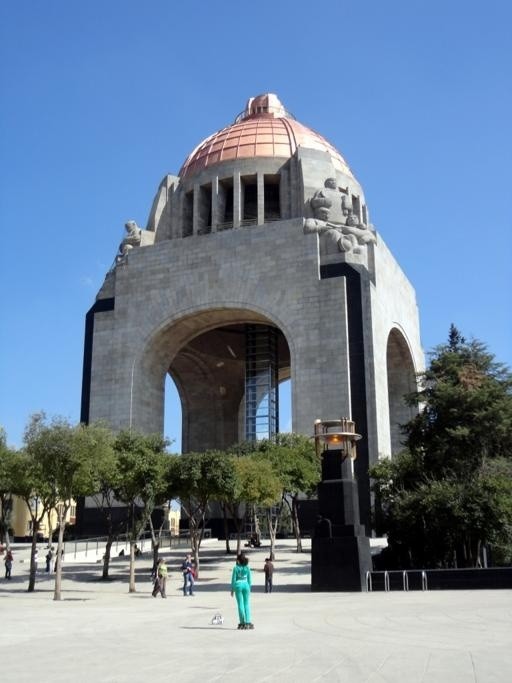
[0,541,4,555]
[115,221,141,265]
[34,544,64,576]
[119,550,126,557]
[135,544,143,558]
[303,177,378,255]
[3,549,13,579]
[264,558,274,593]
[151,557,167,598]
[248,534,256,546]
[230,553,254,630]
[181,553,194,595]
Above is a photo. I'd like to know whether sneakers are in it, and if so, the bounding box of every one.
[236,622,254,630]
